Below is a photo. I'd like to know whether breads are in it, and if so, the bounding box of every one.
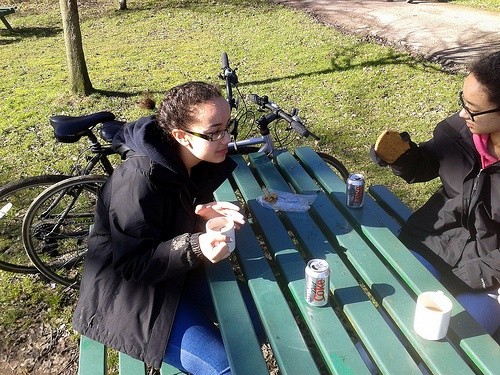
[374,130,411,163]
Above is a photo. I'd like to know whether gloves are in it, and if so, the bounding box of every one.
[439,267,471,298]
[369,131,410,167]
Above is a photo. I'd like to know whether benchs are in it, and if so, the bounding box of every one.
[357,184,415,236]
[78,333,191,375]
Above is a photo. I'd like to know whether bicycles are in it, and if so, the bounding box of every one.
[0,50,348,294]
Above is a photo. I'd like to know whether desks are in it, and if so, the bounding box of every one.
[206,146,500,374]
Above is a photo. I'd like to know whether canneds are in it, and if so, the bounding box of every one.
[345,174,366,208]
[305,259,331,307]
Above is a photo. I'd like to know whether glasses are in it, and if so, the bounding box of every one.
[180,118,236,142]
[458,90,500,122]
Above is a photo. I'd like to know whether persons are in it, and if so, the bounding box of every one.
[355,51,500,375]
[72,81,267,375]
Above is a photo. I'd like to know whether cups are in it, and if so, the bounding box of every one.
[205,216,236,255]
[413,290,453,341]
